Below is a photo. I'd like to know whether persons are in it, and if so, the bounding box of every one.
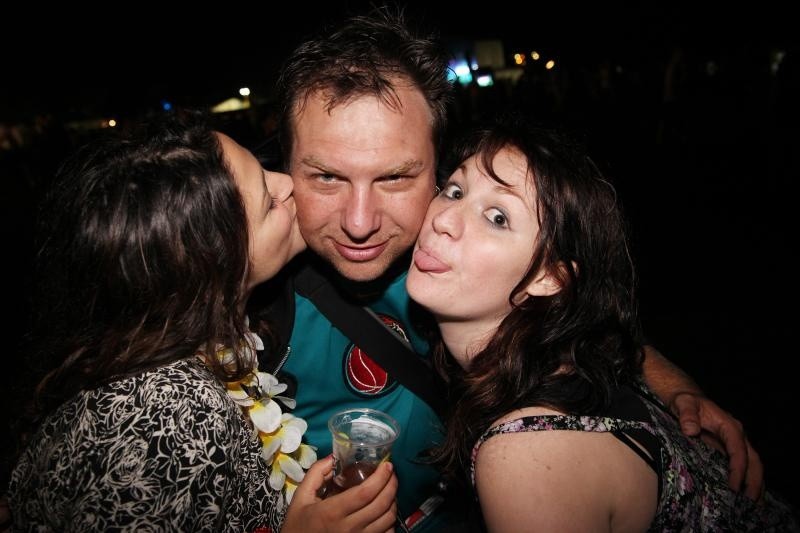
[405,113,800,533]
[244,4,763,533]
[0,111,398,533]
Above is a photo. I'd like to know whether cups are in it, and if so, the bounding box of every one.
[327,408,402,491]
